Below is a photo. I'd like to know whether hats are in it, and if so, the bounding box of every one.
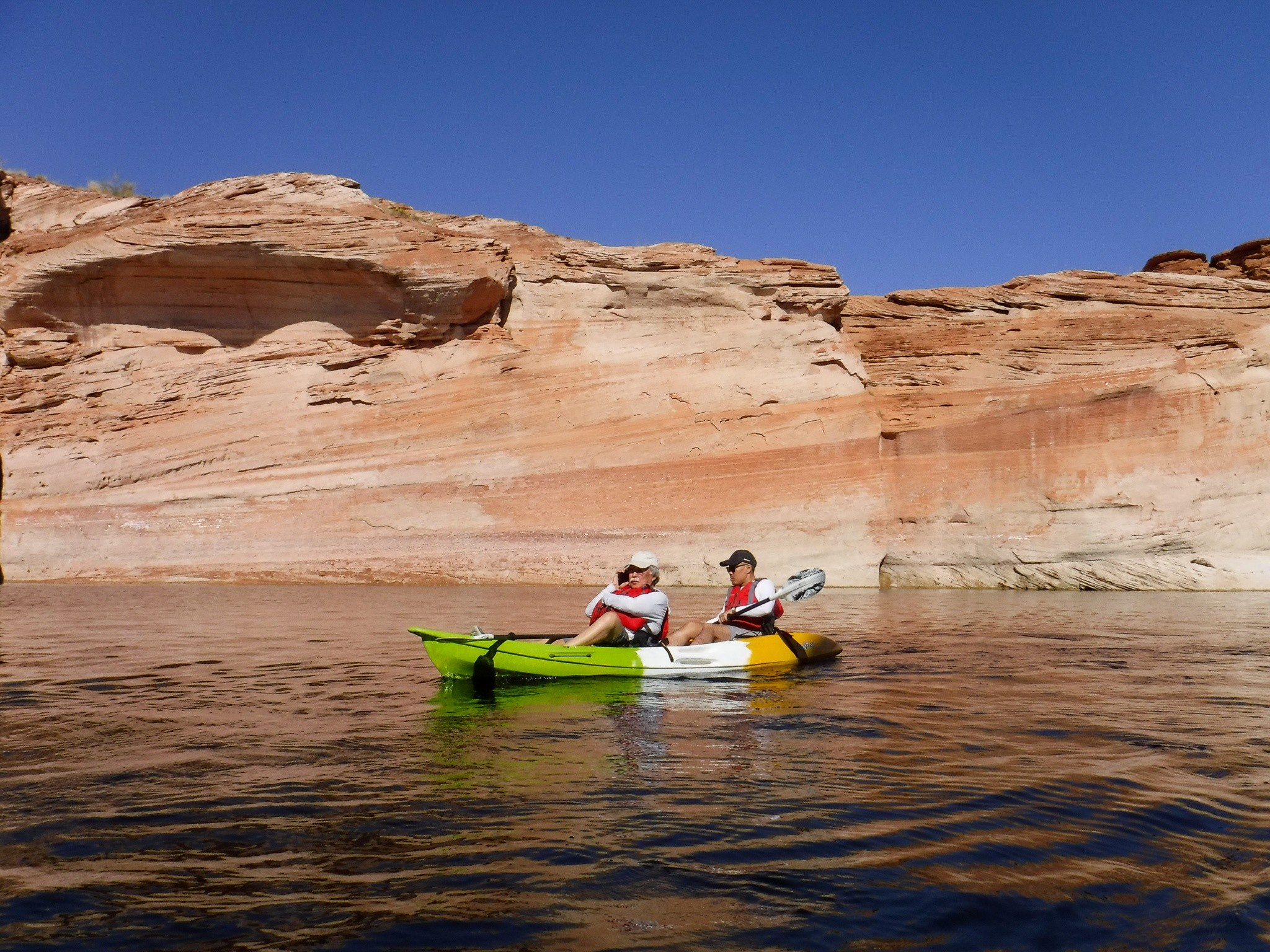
[719,550,757,568]
[624,551,659,570]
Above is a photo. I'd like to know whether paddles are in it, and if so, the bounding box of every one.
[435,632,579,643]
[707,568,826,624]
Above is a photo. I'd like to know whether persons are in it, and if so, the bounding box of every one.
[562,550,671,648]
[657,550,777,646]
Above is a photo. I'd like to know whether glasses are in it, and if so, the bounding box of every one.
[726,564,748,573]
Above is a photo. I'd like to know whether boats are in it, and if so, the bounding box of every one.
[405,622,845,681]
